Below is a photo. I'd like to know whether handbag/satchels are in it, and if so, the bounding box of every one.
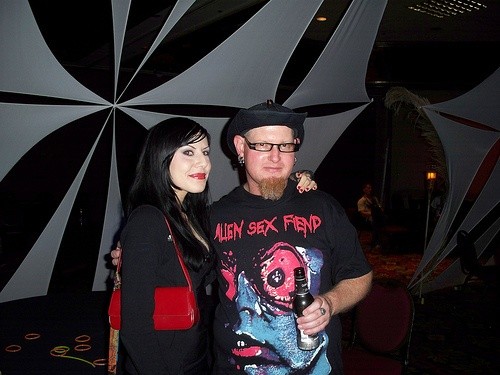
[107,286,200,331]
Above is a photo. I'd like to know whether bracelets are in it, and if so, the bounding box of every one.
[321,294,333,317]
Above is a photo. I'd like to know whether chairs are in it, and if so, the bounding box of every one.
[347,202,377,244]
[384,206,416,248]
[458,231,500,330]
[339,279,416,375]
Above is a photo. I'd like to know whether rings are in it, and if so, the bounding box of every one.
[319,308,326,315]
[315,297,323,306]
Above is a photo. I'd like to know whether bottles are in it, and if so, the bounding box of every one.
[293,267,320,351]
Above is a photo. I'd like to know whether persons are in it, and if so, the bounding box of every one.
[110,99,373,375]
[108,116,318,375]
[357,183,419,252]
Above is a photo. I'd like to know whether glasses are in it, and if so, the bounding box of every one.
[244,136,299,152]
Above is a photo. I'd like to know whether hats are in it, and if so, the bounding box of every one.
[227,103,308,156]
